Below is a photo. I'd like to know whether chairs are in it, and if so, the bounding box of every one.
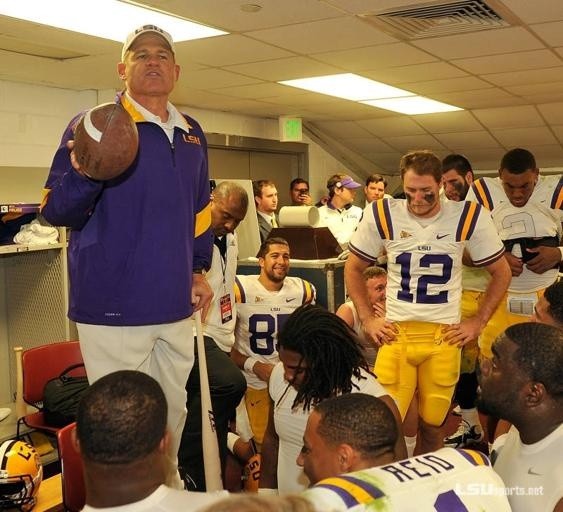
[14,341,86,440]
[57,423,85,512]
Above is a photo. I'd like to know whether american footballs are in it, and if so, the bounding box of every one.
[74,103,140,180]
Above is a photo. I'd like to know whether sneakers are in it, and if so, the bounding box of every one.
[452,405,461,416]
[443,420,483,448]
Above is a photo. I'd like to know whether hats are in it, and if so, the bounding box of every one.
[121,24,176,63]
[328,177,362,189]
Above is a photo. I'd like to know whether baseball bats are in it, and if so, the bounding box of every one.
[194,294,223,491]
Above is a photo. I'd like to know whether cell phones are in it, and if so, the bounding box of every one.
[300,189,307,199]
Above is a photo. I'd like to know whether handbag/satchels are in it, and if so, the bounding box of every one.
[43,364,90,428]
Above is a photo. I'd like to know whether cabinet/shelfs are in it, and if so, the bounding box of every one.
[0,226,71,445]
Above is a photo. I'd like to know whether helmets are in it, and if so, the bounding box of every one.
[0,438,44,512]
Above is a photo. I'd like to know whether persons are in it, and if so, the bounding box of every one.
[40,24,215,494]
[73,148,562,512]
